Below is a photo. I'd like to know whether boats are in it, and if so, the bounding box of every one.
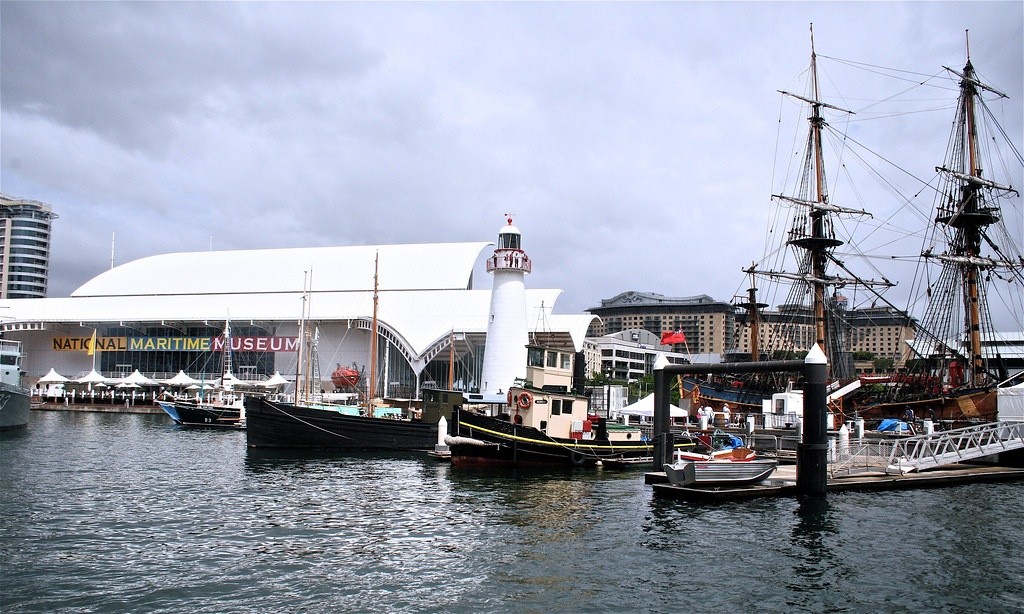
[0,306,31,430]
[155,23,1024,483]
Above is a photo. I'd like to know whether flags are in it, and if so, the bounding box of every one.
[660,329,685,345]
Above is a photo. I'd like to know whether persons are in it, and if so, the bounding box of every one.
[626,433,634,441]
[61,394,67,406]
[591,432,593,439]
[925,406,937,424]
[903,405,911,420]
[909,408,915,419]
[734,417,739,425]
[415,407,422,418]
[621,414,626,424]
[191,395,243,408]
[738,417,745,429]
[618,418,622,423]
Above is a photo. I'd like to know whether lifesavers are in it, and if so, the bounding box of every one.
[518,392,532,408]
[507,391,512,407]
